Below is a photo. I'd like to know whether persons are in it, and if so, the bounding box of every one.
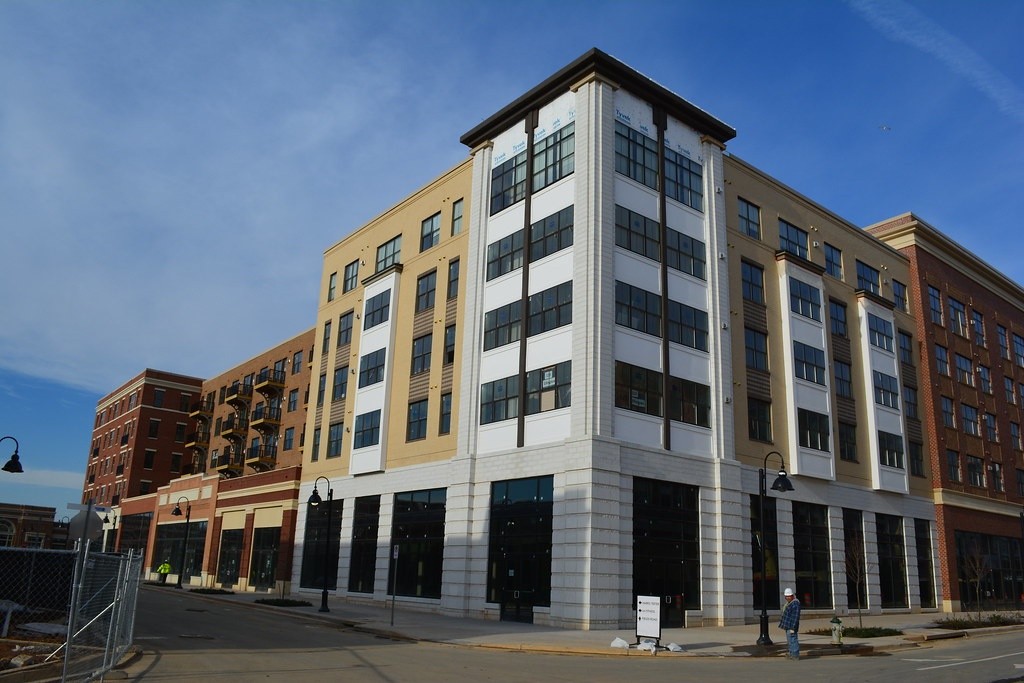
[156,560,171,583]
[778,588,801,660]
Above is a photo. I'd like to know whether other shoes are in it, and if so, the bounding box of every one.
[787,656,799,662]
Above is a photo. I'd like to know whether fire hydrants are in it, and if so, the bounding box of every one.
[829,614,844,645]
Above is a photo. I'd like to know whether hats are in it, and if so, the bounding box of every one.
[165,560,167,562]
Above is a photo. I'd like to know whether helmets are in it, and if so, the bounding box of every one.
[784,588,793,596]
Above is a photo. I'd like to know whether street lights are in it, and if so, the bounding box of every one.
[756,449,796,646]
[61,514,70,549]
[308,475,334,613]
[102,508,117,554]
[170,496,191,590]
